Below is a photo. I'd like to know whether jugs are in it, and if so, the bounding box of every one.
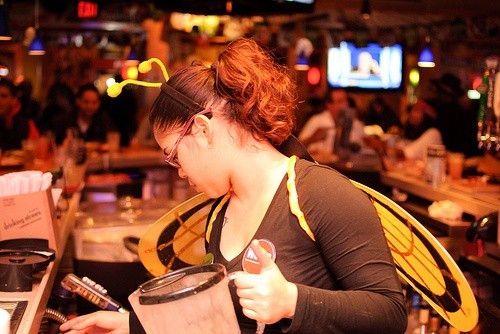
[127,263,266,334]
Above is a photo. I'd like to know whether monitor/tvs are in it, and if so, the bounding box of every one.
[327,43,405,93]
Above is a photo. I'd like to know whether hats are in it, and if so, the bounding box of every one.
[431,73,466,99]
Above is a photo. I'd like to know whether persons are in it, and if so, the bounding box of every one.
[0,72,140,153]
[59,38,408,334]
[418,73,486,177]
[299,86,394,196]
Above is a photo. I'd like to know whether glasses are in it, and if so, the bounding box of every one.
[164,107,212,167]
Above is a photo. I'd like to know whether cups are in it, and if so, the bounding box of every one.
[426,144,447,184]
[448,153,463,181]
[434,160,450,192]
